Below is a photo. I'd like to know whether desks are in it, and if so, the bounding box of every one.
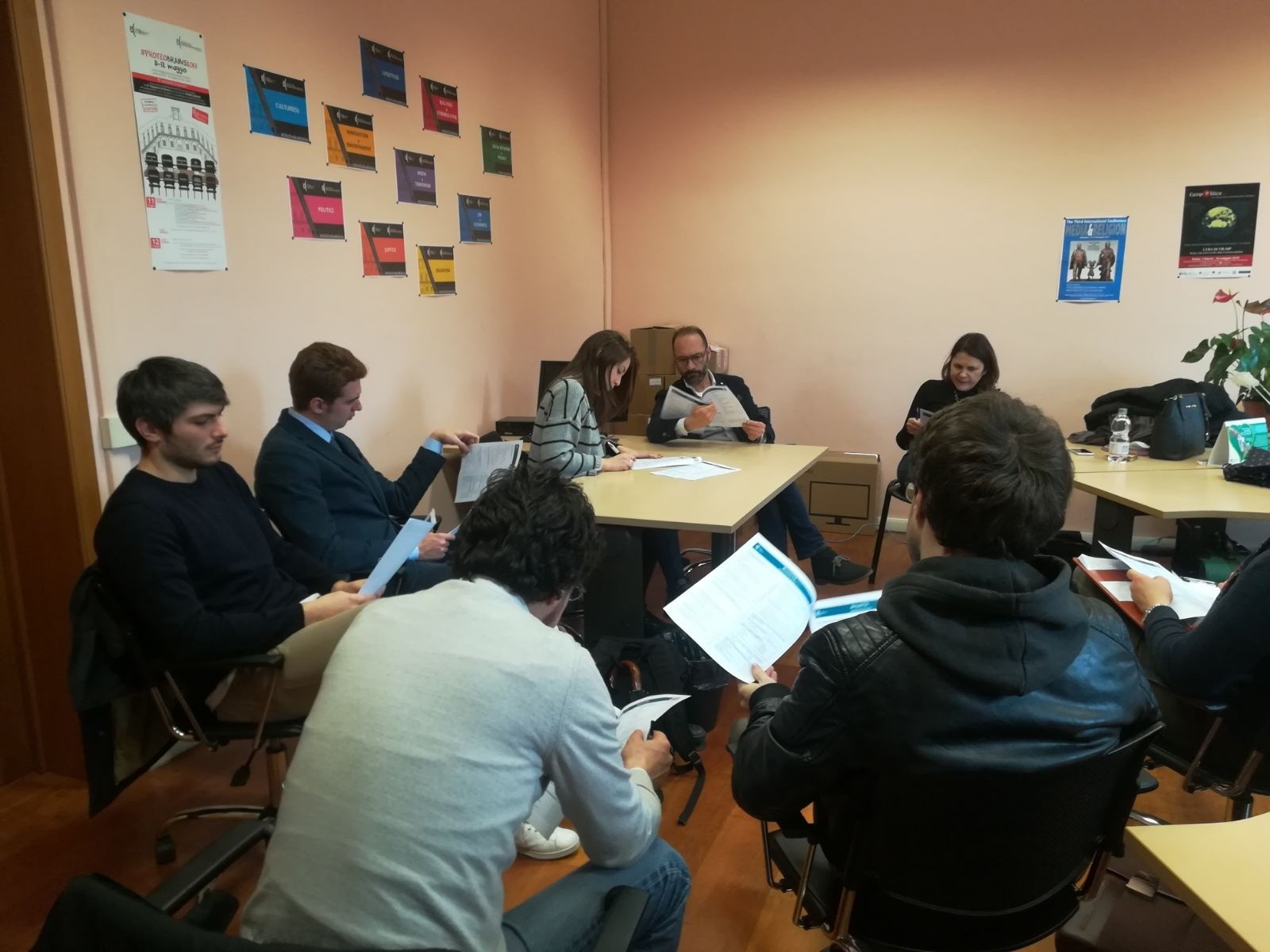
[575,430,828,573]
[1065,434,1270,575]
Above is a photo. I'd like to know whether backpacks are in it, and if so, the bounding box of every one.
[584,629,699,772]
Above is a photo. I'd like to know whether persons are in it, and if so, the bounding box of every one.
[896,333,999,488]
[1127,540,1270,778]
[224,466,690,952]
[527,330,637,478]
[95,342,481,731]
[645,326,874,586]
[730,394,1159,952]
[1071,240,1115,281]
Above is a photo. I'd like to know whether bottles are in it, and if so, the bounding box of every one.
[1108,408,1131,465]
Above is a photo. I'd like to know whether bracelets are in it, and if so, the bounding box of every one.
[1142,604,1170,623]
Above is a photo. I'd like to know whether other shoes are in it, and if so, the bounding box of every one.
[662,579,695,619]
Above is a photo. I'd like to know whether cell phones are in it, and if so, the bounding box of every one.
[1068,448,1094,455]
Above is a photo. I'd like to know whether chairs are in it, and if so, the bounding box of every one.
[89,564,303,820]
[251,495,444,600]
[1148,675,1270,797]
[1126,810,1270,952]
[757,779,1109,952]
[33,805,281,952]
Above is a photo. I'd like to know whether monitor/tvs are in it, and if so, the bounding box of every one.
[537,361,569,414]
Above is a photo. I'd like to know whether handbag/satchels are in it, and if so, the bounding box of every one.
[1148,391,1213,461]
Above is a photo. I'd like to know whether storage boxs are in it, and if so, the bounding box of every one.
[628,324,730,437]
[795,451,882,539]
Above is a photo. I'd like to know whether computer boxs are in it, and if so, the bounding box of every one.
[496,416,535,436]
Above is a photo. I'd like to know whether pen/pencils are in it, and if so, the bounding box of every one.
[608,441,620,455]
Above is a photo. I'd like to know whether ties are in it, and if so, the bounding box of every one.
[330,437,342,453]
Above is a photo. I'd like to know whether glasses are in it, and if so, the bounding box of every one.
[675,347,708,366]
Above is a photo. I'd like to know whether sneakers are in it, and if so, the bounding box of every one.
[811,546,874,586]
[515,818,580,860]
